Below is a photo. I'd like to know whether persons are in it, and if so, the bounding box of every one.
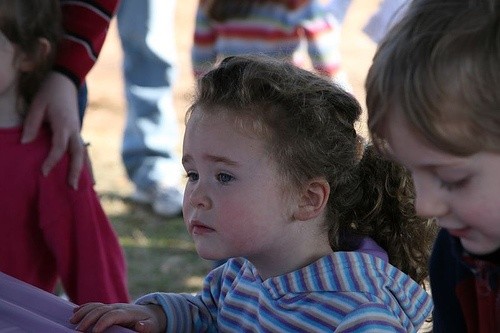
[192,0,353,101]
[1,1,186,218]
[0,0,131,306]
[66,52,434,333]
[365,0,500,333]
